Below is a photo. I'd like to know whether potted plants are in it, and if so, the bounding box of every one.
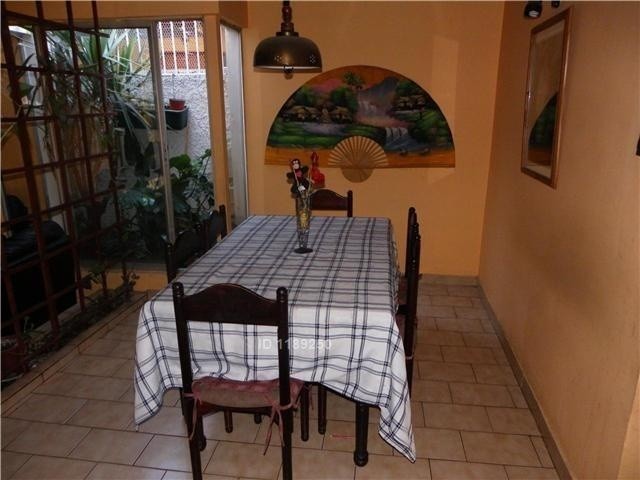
[1,314,46,378]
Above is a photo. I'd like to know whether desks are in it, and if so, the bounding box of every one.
[135,216,417,467]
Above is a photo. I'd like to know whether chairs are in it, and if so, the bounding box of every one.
[398,207,417,309]
[320,224,420,435]
[164,221,208,284]
[172,281,310,480]
[311,188,353,217]
[207,205,228,248]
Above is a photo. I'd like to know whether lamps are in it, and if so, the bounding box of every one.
[253,0,322,79]
[525,2,543,18]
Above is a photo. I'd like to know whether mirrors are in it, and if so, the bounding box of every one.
[520,6,574,189]
